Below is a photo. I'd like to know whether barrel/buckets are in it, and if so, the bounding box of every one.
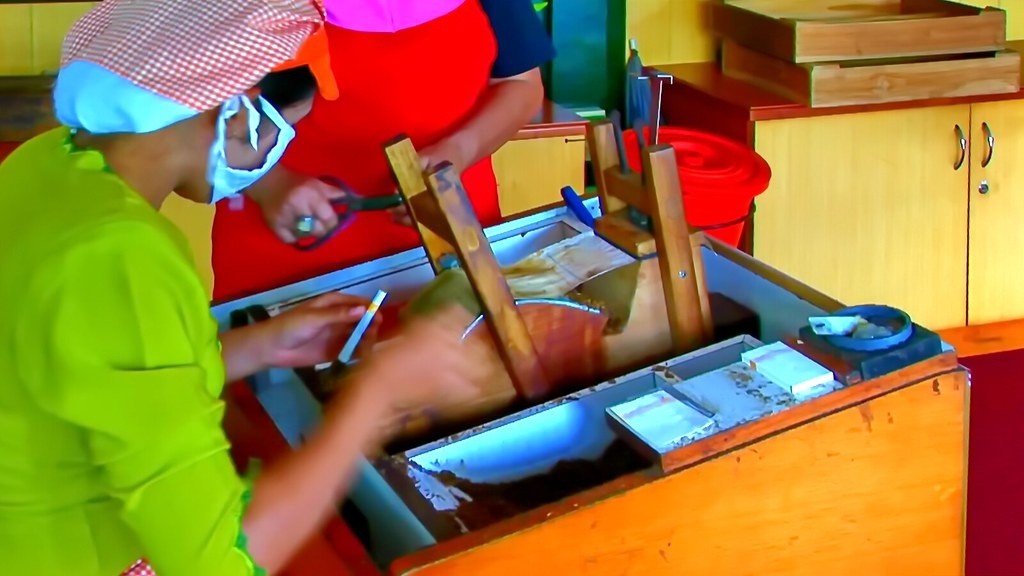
[620,123,773,256]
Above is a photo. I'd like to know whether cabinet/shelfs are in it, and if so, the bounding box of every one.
[661,87,1024,359]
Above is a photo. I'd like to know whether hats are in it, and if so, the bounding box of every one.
[269,23,341,103]
[51,61,199,134]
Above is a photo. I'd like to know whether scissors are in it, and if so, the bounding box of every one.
[292,173,404,252]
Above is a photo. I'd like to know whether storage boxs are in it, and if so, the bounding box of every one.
[712,0,1021,109]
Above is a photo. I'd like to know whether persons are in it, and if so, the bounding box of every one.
[212,0,559,310]
[0,0,509,576]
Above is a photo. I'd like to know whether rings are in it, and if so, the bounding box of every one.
[297,216,316,234]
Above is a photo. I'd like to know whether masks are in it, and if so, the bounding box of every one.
[197,94,295,205]
